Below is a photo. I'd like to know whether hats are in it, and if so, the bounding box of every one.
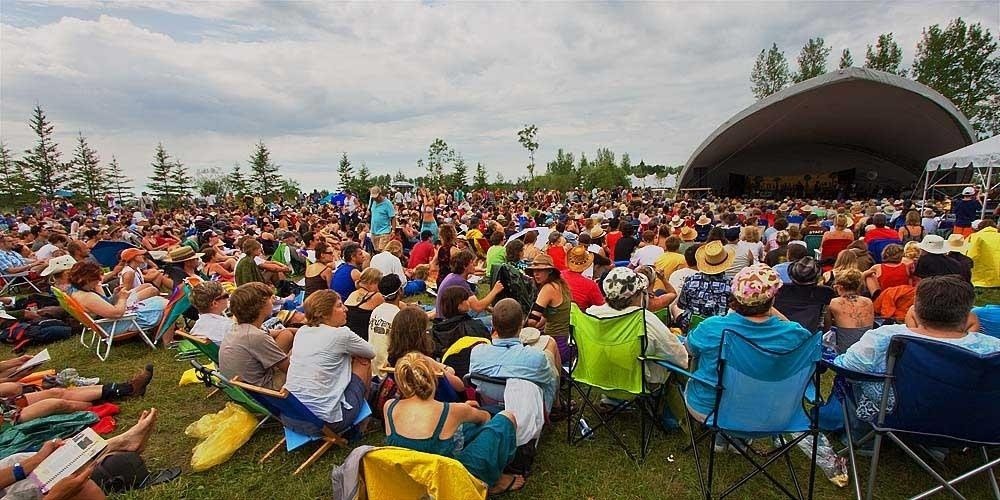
[91,451,182,496]
[136,217,148,222]
[370,186,381,198]
[162,245,206,263]
[917,234,970,254]
[40,255,77,277]
[695,240,821,306]
[884,206,896,214]
[107,225,120,235]
[121,248,147,262]
[638,215,712,240]
[526,226,607,272]
[602,266,649,300]
[779,204,813,212]
[962,187,976,195]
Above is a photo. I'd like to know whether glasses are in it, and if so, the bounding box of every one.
[381,288,400,300]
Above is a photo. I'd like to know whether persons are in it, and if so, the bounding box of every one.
[0,354,159,500]
[0,177,999,383]
[833,273,1000,471]
[281,288,377,442]
[384,350,526,496]
[217,280,299,392]
[469,298,580,449]
[685,262,813,457]
[587,266,690,437]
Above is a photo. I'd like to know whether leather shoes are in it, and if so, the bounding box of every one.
[829,433,874,487]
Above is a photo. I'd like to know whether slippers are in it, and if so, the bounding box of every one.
[495,475,526,494]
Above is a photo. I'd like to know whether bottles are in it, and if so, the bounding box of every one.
[820,325,838,375]
[453,424,465,451]
[774,434,789,458]
[578,418,595,440]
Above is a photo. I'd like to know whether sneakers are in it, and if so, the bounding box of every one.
[710,435,752,455]
[130,365,153,396]
[599,398,634,414]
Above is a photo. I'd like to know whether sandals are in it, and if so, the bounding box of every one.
[550,400,579,422]
